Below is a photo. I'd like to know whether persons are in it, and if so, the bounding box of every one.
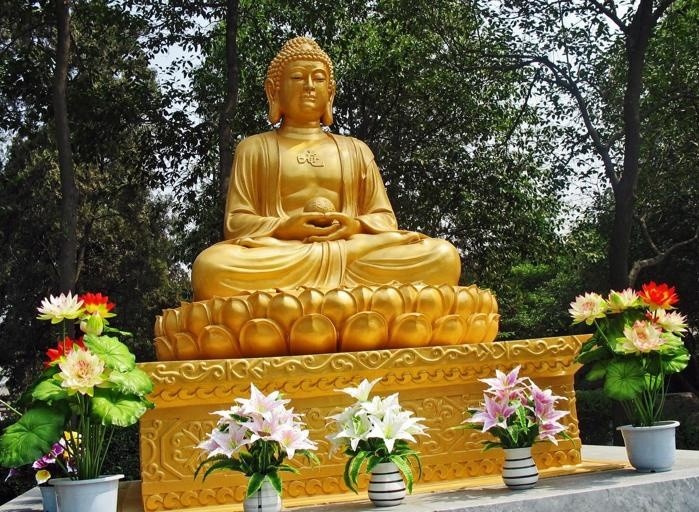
[191,35,462,302]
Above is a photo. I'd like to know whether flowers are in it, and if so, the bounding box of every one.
[323,376,433,497]
[190,381,320,497]
[567,280,691,427]
[0,291,156,485]
[444,363,578,456]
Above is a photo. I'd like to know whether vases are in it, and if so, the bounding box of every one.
[367,461,409,508]
[40,474,126,512]
[243,473,283,512]
[616,420,681,473]
[501,445,539,491]
